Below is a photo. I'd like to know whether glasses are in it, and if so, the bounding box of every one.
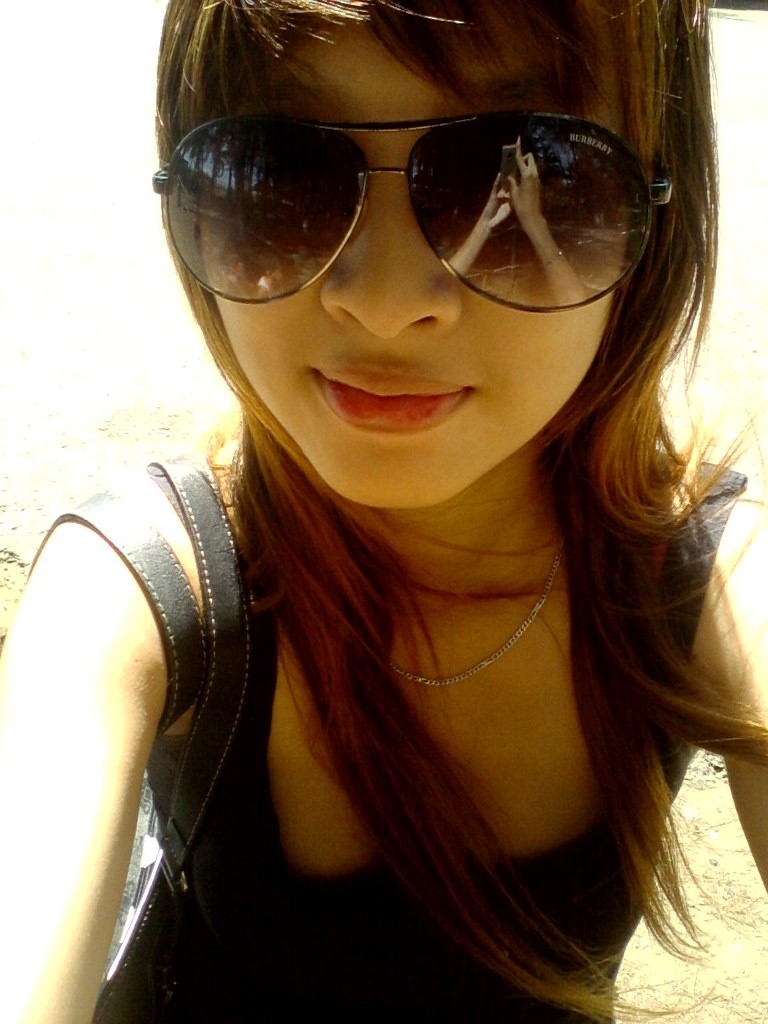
[154,109,675,314]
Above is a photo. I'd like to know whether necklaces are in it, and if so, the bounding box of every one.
[388,534,563,688]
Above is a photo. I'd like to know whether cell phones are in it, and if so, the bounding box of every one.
[497,144,518,199]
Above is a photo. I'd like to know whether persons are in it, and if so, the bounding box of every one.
[0,0,768,1024]
[448,134,587,306]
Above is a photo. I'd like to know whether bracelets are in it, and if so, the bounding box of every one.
[542,248,564,268]
